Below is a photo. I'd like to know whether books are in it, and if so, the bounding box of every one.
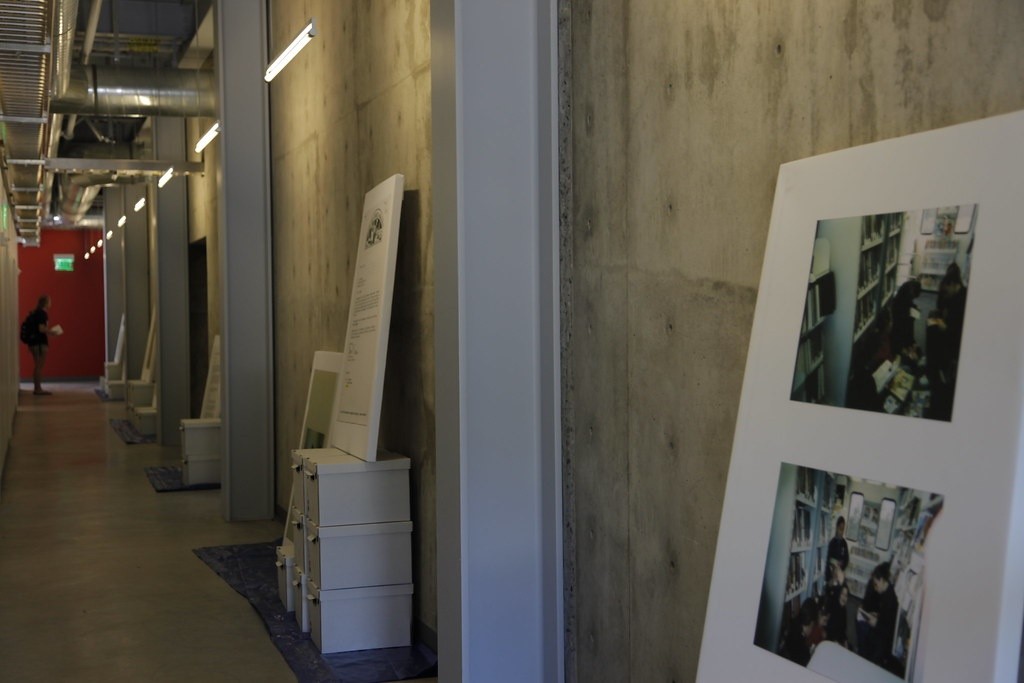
[784,465,844,602]
[791,212,903,402]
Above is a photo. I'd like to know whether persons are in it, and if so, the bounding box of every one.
[822,559,848,643]
[825,516,849,587]
[892,280,921,349]
[20,295,57,395]
[935,277,950,316]
[855,561,899,666]
[783,597,831,667]
[939,262,967,361]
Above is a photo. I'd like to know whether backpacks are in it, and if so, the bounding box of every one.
[20,312,33,345]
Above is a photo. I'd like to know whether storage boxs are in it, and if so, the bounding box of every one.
[278,441,414,654]
[99,362,158,436]
[179,418,224,488]
